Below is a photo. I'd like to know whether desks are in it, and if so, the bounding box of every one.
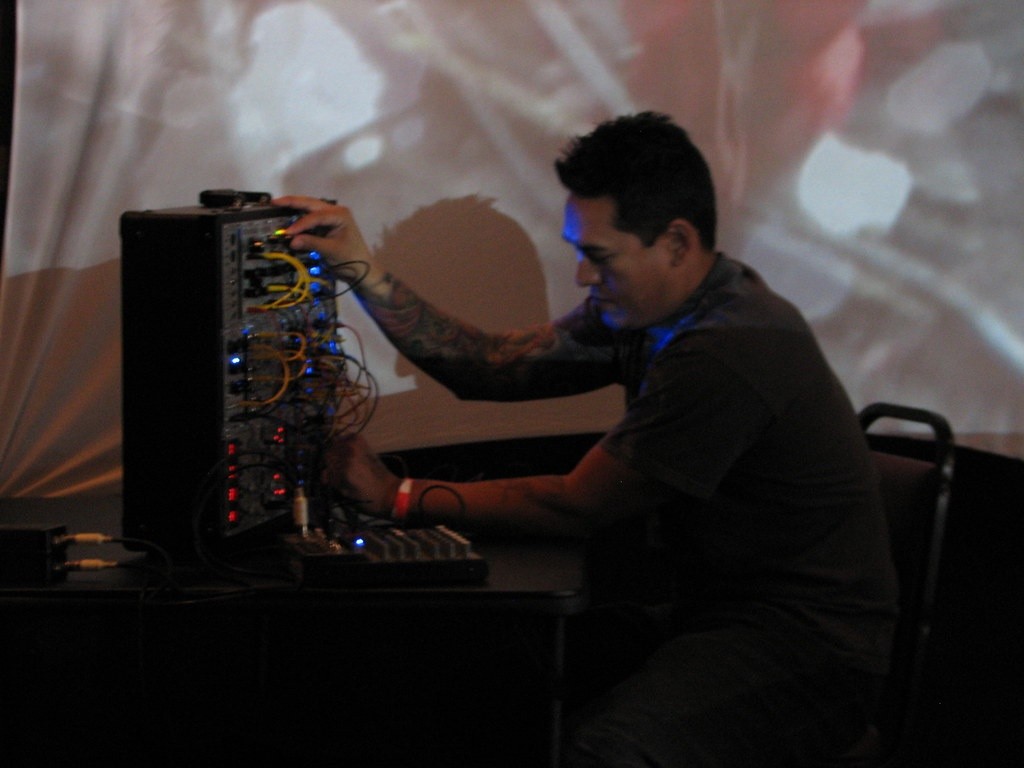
[0,491,593,768]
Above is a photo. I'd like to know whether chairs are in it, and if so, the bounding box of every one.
[833,403,956,768]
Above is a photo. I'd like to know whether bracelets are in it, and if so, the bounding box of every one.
[390,477,413,518]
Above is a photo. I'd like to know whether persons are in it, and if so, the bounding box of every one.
[268,110,901,768]
